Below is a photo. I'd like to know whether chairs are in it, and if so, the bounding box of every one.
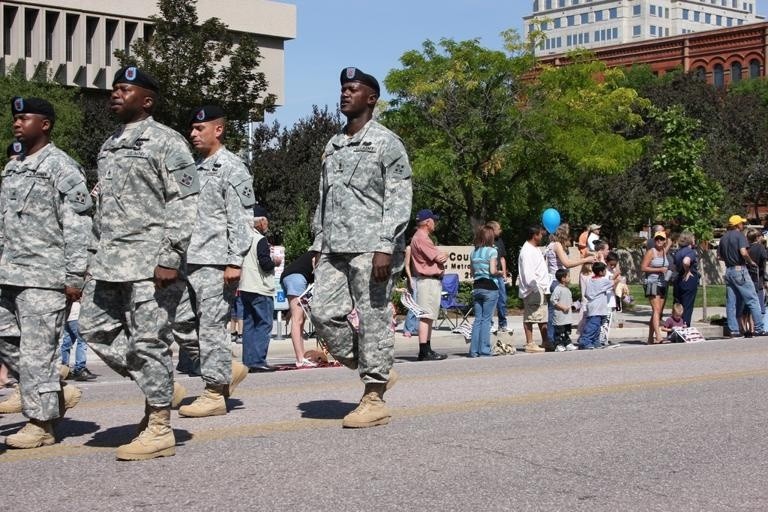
[433,273,473,332]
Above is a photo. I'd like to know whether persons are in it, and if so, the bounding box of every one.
[0,96,93,449]
[171,104,256,417]
[310,66,413,428]
[76,65,200,460]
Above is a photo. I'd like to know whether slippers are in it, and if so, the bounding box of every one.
[652,337,672,346]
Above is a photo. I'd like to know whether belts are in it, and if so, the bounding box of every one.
[422,274,444,281]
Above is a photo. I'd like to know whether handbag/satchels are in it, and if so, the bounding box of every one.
[491,340,518,357]
[644,282,659,298]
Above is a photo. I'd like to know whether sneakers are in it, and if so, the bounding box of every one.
[295,357,318,369]
[385,369,401,391]
[73,368,99,381]
[731,327,768,340]
[523,341,610,354]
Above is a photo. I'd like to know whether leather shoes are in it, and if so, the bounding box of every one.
[416,351,448,361]
[251,362,281,375]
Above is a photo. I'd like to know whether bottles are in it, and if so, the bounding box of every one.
[683,321,687,336]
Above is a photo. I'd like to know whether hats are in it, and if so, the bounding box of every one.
[339,66,381,98]
[9,96,56,118]
[111,66,161,95]
[6,140,26,158]
[728,214,747,227]
[254,207,269,217]
[415,208,440,221]
[188,104,226,125]
[654,231,667,239]
[589,223,602,231]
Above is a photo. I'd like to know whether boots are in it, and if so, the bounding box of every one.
[223,359,249,398]
[0,383,23,413]
[178,385,227,418]
[342,383,392,429]
[5,419,57,449]
[58,382,82,416]
[114,407,176,462]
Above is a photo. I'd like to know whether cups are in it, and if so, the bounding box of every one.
[570,303,578,312]
[622,296,630,304]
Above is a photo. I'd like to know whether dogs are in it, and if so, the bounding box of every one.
[303,349,328,364]
